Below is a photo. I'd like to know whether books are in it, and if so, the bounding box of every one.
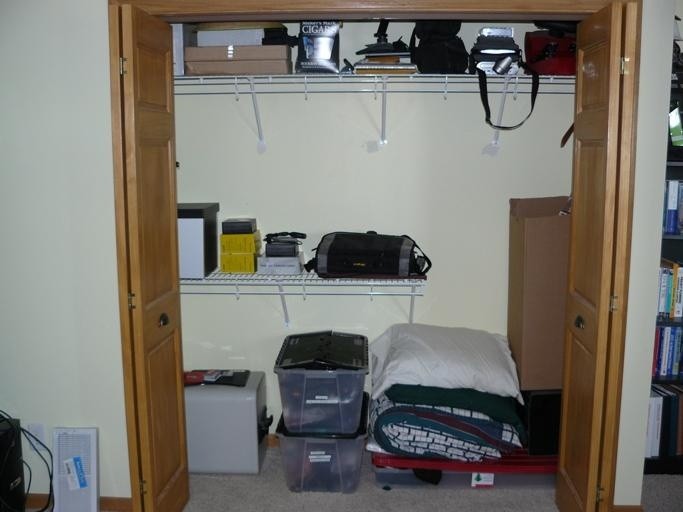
[644,180,683,459]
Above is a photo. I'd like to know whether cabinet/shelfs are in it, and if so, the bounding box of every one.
[171,71,580,325]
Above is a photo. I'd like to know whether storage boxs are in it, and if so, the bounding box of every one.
[500,196,572,391]
[271,330,371,496]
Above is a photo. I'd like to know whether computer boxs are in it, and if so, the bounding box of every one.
[0,416,26,511]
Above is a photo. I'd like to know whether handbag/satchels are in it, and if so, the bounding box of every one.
[472,35,520,62]
[315,232,431,278]
